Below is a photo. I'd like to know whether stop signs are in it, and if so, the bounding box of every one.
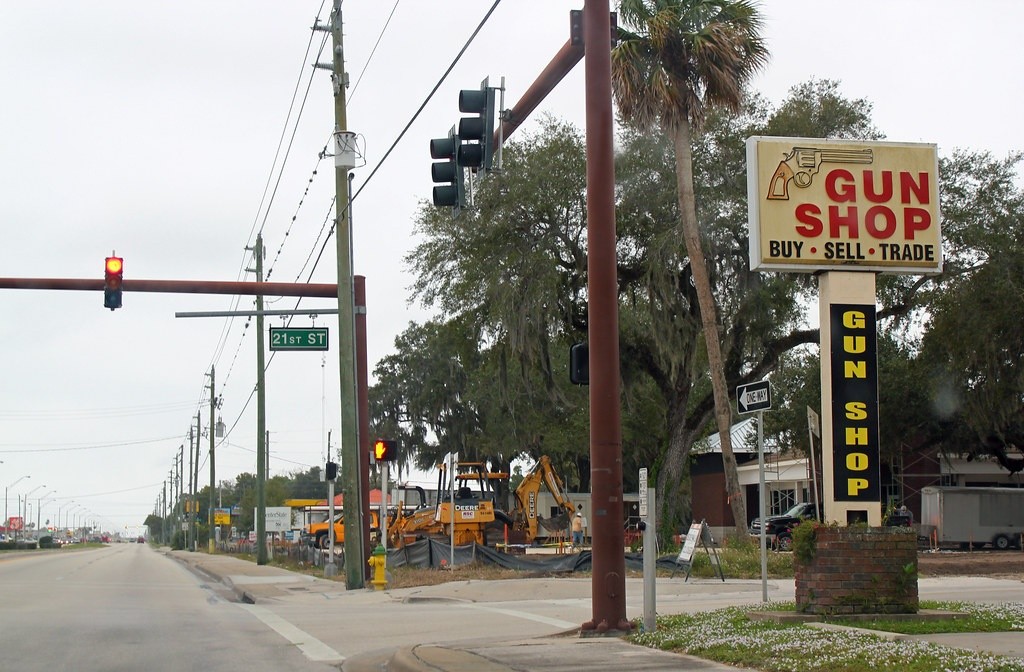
[747,135,945,275]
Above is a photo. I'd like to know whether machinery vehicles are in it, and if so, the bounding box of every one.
[299,454,576,552]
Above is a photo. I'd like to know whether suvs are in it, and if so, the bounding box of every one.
[750,503,823,551]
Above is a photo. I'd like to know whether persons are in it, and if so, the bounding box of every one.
[571,512,588,552]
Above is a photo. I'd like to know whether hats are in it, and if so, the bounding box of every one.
[577,513,581,516]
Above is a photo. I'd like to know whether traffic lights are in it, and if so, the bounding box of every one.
[104,257,124,308]
[429,125,469,219]
[376,440,396,460]
[458,86,495,172]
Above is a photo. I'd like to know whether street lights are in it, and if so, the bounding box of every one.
[59,500,103,536]
[37,490,57,548]
[5,476,46,541]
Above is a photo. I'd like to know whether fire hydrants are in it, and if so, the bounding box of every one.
[367,543,388,591]
